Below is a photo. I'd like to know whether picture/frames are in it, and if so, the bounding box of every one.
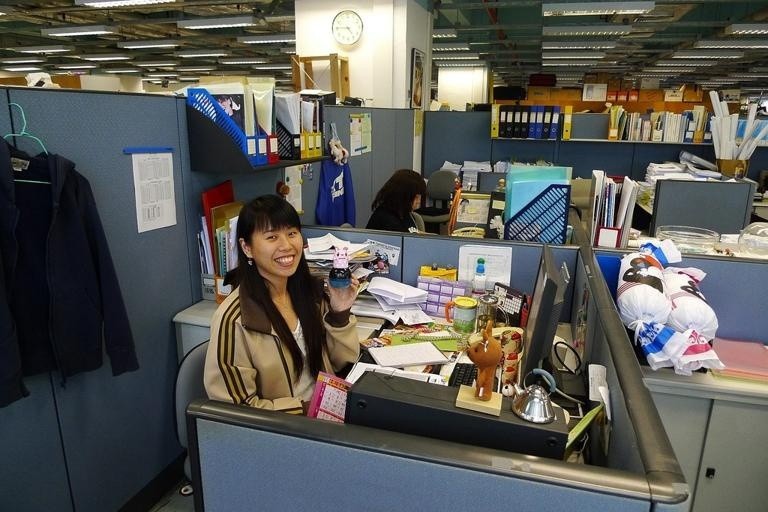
[410,48,426,109]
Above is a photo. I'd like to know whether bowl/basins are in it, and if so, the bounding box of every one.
[655,224,720,255]
[714,158,751,180]
[738,222,767,258]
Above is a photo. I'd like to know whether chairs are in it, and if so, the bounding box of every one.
[413,167,461,233]
[171,331,220,483]
[410,209,427,231]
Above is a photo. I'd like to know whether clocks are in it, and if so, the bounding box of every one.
[328,9,367,47]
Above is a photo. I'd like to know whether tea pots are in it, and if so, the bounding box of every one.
[510,369,557,423]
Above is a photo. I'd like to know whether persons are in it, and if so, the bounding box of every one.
[203,192,360,422]
[365,169,427,232]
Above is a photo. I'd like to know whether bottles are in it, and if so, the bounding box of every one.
[329,247,352,288]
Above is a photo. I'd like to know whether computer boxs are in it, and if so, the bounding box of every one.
[344,370,568,462]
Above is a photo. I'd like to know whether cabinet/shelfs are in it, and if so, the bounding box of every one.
[169,292,385,365]
[639,354,768,510]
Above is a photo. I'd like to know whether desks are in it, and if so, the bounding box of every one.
[346,312,589,465]
[634,193,654,219]
[754,196,768,222]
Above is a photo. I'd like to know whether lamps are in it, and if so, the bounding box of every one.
[543,41,617,49]
[725,24,768,35]
[431,53,479,60]
[626,74,680,87]
[1,0,295,80]
[432,29,458,38]
[543,26,632,36]
[430,78,439,93]
[432,42,469,51]
[694,40,767,49]
[695,67,767,89]
[542,52,606,59]
[542,1,655,18]
[670,52,745,58]
[489,71,508,88]
[435,61,487,67]
[543,61,599,66]
[643,61,719,71]
[556,71,584,87]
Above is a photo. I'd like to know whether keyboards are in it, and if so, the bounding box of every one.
[449,362,498,392]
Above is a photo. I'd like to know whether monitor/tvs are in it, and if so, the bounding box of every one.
[518,244,565,396]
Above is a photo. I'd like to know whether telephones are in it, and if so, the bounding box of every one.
[467,327,524,347]
[450,226,486,238]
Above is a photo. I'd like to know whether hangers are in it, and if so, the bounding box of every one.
[0,98,54,185]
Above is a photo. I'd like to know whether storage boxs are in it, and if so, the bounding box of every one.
[713,155,750,178]
[492,71,742,104]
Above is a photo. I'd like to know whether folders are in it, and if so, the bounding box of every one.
[490,103,573,141]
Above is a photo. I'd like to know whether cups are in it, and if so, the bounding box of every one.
[444,297,477,333]
[472,273,486,293]
[477,292,509,333]
